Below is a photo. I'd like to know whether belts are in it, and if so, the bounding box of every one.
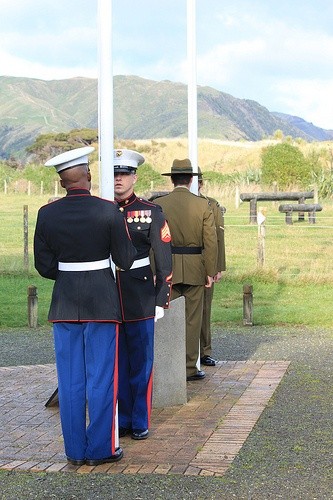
[202,244,204,250]
[86,447,123,465]
[171,246,202,254]
[58,258,110,271]
[115,256,150,271]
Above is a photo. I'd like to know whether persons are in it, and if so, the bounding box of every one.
[198,166,226,366]
[34,145,137,464]
[150,159,219,381]
[114,148,174,441]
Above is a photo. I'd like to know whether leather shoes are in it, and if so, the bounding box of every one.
[66,456,86,465]
[131,428,149,439]
[201,355,215,366]
[119,428,132,437]
[186,371,205,381]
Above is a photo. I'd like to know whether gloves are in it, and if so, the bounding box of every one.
[154,305,164,321]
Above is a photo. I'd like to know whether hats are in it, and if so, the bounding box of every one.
[113,149,145,171]
[44,147,95,172]
[161,159,202,176]
[198,167,210,180]
